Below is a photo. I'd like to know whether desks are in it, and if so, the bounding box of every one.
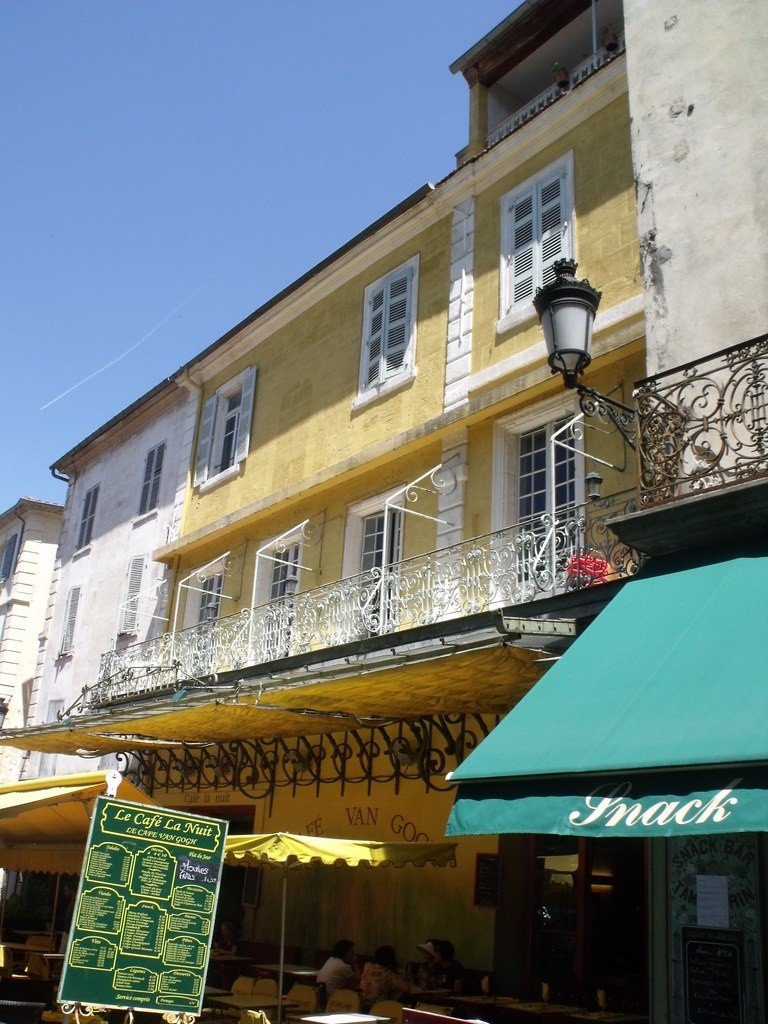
[0,943,648,1024]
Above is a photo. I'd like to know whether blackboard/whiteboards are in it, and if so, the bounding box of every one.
[682,927,746,1024]
[473,852,503,909]
[175,857,220,884]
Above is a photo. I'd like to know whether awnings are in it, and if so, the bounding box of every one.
[444,528,768,838]
[0,769,163,948]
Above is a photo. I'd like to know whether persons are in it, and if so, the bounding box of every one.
[316,939,364,1010]
[360,945,422,1013]
[213,921,241,956]
[60,880,78,933]
[412,939,466,994]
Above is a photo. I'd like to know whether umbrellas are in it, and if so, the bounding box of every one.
[222,832,458,1024]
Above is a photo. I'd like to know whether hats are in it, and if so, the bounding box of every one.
[416,941,436,958]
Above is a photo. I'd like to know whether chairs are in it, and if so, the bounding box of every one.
[286,984,318,1012]
[231,976,254,994]
[368,1000,404,1024]
[325,990,359,1013]
[252,978,278,1020]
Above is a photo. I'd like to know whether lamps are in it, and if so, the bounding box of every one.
[205,602,218,620]
[284,575,297,595]
[172,685,234,702]
[532,259,680,498]
